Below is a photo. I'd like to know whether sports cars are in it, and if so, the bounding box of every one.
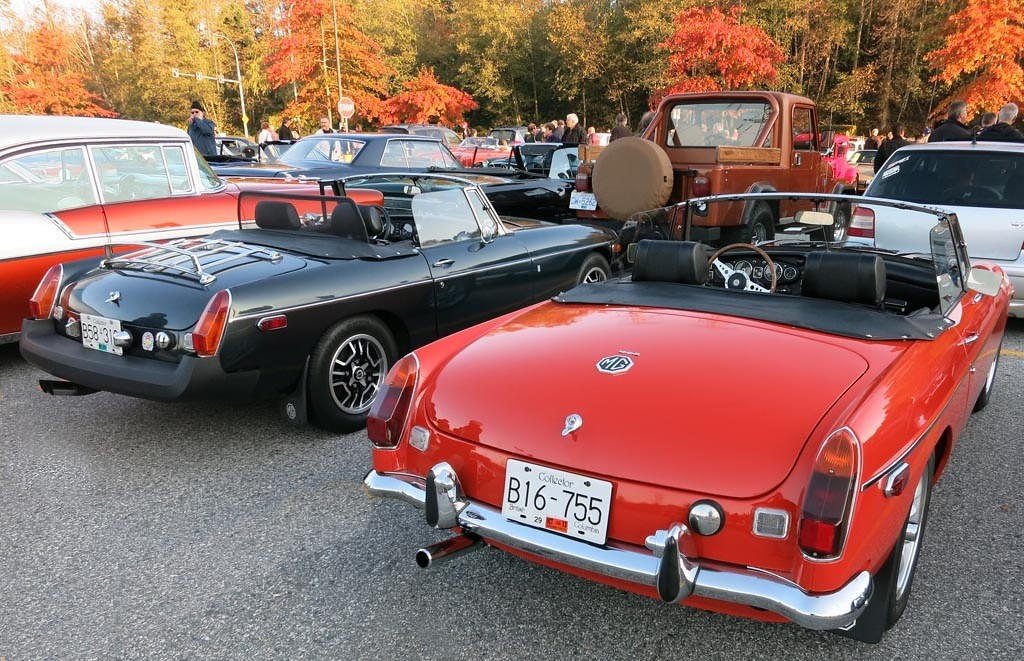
[838,140,1024,323]
[17,169,623,435]
[361,191,1017,644]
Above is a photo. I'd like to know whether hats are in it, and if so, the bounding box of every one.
[926,128,931,133]
[191,101,203,112]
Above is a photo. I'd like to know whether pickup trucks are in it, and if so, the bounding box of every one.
[570,88,859,262]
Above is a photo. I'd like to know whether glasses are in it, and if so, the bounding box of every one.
[191,111,203,114]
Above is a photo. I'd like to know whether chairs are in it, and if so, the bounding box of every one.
[253,201,301,232]
[632,239,710,287]
[329,202,384,243]
[925,154,973,202]
[801,249,886,308]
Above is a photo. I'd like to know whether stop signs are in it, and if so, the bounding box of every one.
[338,97,355,119]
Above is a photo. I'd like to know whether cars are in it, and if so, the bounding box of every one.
[0,113,380,352]
[113,122,885,222]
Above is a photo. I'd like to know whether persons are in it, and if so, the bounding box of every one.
[524,112,601,145]
[608,114,632,142]
[461,123,494,143]
[187,101,217,156]
[864,100,1023,201]
[635,110,742,149]
[257,115,366,161]
